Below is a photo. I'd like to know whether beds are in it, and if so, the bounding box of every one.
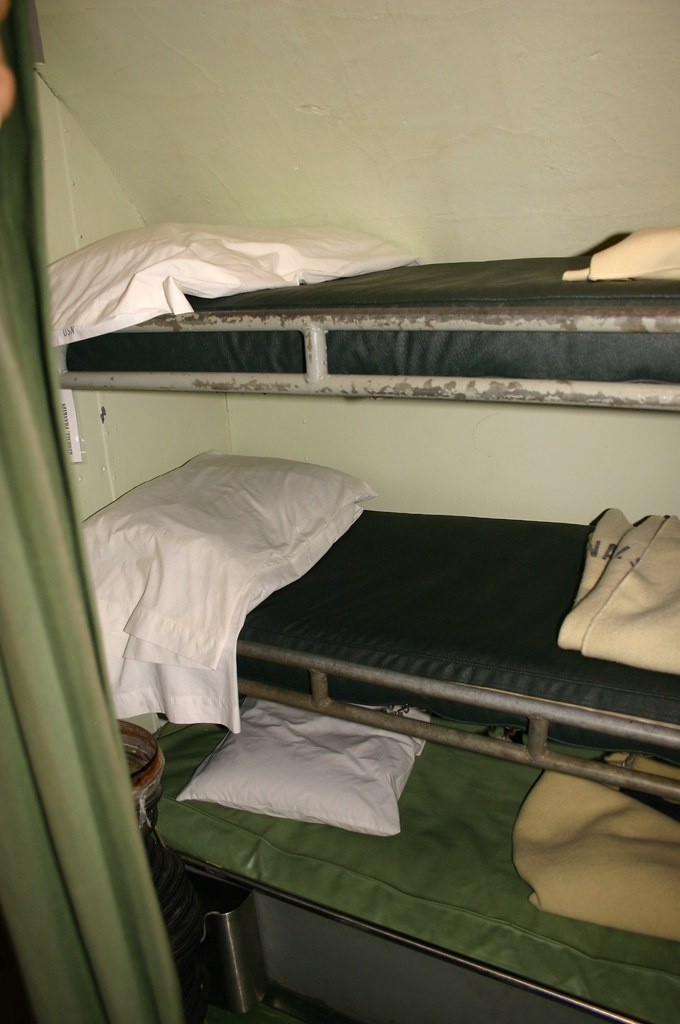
[43,222,680,1024]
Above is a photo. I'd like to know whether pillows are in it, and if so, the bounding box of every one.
[174,703,426,839]
[45,225,426,347]
[79,447,377,734]
